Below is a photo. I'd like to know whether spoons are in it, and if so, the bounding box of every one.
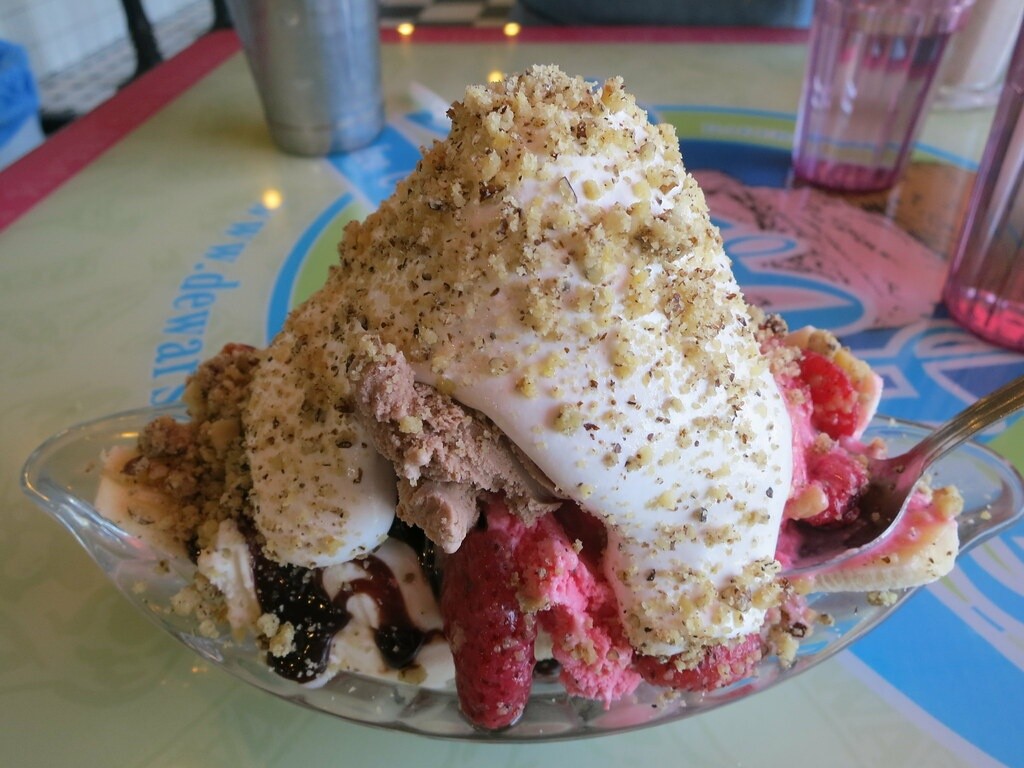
[773,372,1024,580]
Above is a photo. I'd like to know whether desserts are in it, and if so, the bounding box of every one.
[83,65,964,726]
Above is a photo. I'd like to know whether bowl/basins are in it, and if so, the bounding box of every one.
[19,403,1024,746]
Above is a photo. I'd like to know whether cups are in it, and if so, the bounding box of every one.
[788,0,974,198]
[937,10,1024,354]
[222,0,386,159]
